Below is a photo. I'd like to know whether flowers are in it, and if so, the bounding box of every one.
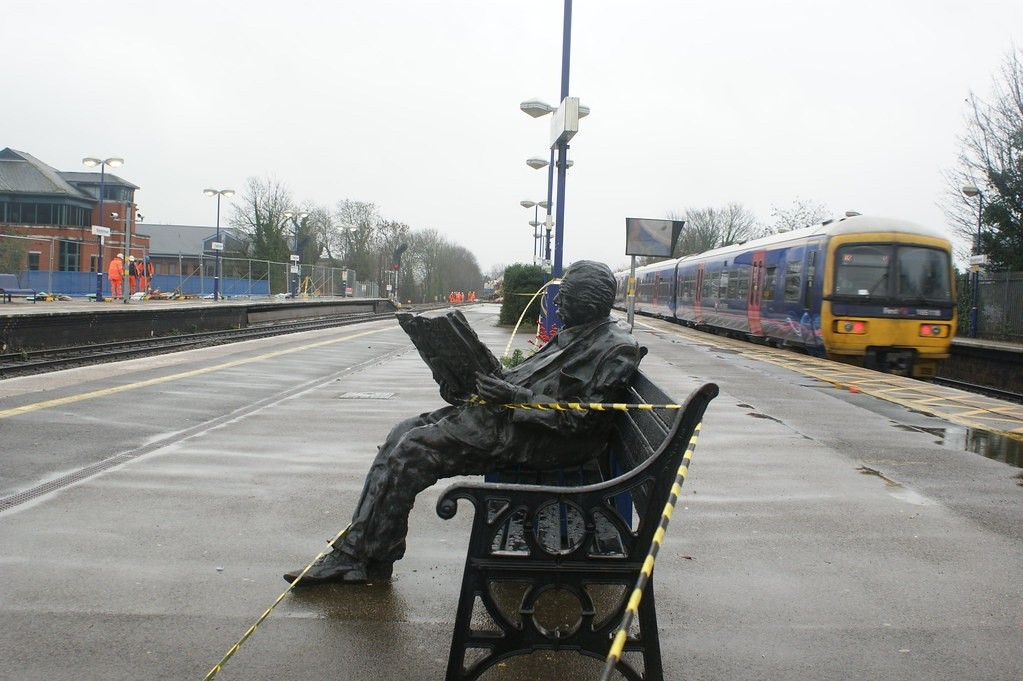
[528,321,558,348]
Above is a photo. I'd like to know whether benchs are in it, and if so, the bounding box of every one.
[436,345,719,681]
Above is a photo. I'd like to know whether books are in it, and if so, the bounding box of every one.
[392,307,504,403]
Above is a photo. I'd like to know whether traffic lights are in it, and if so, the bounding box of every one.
[394,251,400,270]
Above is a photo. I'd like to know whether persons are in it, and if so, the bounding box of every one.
[129,256,139,295]
[284,260,646,584]
[139,256,154,292]
[449,291,464,302]
[108,253,125,299]
[468,291,475,302]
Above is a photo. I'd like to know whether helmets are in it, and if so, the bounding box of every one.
[117,254,123,259]
[129,256,134,261]
[145,256,150,262]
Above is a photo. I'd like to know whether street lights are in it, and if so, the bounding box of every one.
[527,158,573,272]
[203,190,235,297]
[520,98,590,271]
[284,209,310,298]
[82,157,125,301]
[336,224,358,297]
[609,212,958,380]
[529,221,553,267]
[521,200,550,267]
[961,186,982,336]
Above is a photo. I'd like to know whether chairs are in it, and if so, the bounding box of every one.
[0,274,36,305]
